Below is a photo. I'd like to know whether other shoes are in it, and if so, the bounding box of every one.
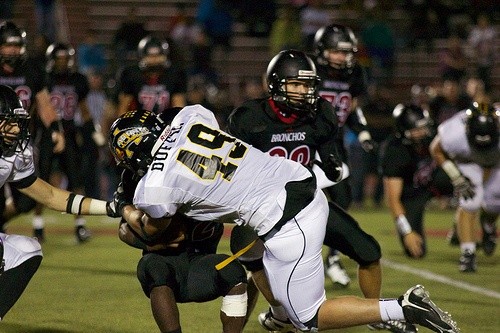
[458,252,475,272]
[33,228,44,242]
[75,224,87,242]
[482,224,495,256]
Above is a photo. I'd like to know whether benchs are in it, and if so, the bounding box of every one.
[61,0,473,81]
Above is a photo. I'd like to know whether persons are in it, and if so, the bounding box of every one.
[108,105,463,333]
[0,85,119,322]
[0,1,500,333]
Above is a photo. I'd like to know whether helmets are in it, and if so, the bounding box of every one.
[46,42,75,70]
[107,109,170,180]
[0,84,32,156]
[139,36,167,58]
[0,22,27,68]
[392,103,434,146]
[466,106,500,150]
[266,49,319,113]
[314,25,358,74]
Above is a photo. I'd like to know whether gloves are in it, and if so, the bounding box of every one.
[442,159,475,201]
[106,182,129,219]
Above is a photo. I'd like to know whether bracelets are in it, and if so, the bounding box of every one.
[395,215,412,235]
[90,199,106,216]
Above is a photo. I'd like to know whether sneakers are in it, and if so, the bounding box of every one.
[258,307,303,333]
[323,254,350,287]
[367,320,417,333]
[396,284,459,333]
